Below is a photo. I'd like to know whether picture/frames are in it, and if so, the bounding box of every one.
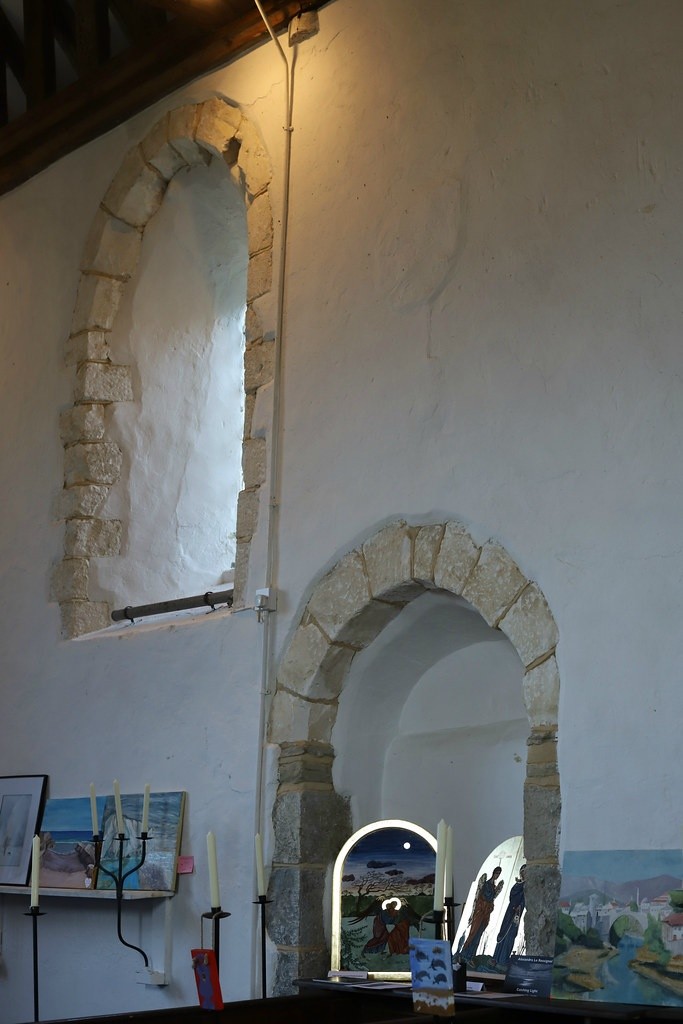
[0,774,48,887]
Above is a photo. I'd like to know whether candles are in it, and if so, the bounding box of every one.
[141,783,151,832]
[113,778,124,835]
[31,834,40,907]
[90,782,99,836]
[444,824,454,897]
[207,830,220,907]
[254,831,265,896]
[433,818,444,910]
